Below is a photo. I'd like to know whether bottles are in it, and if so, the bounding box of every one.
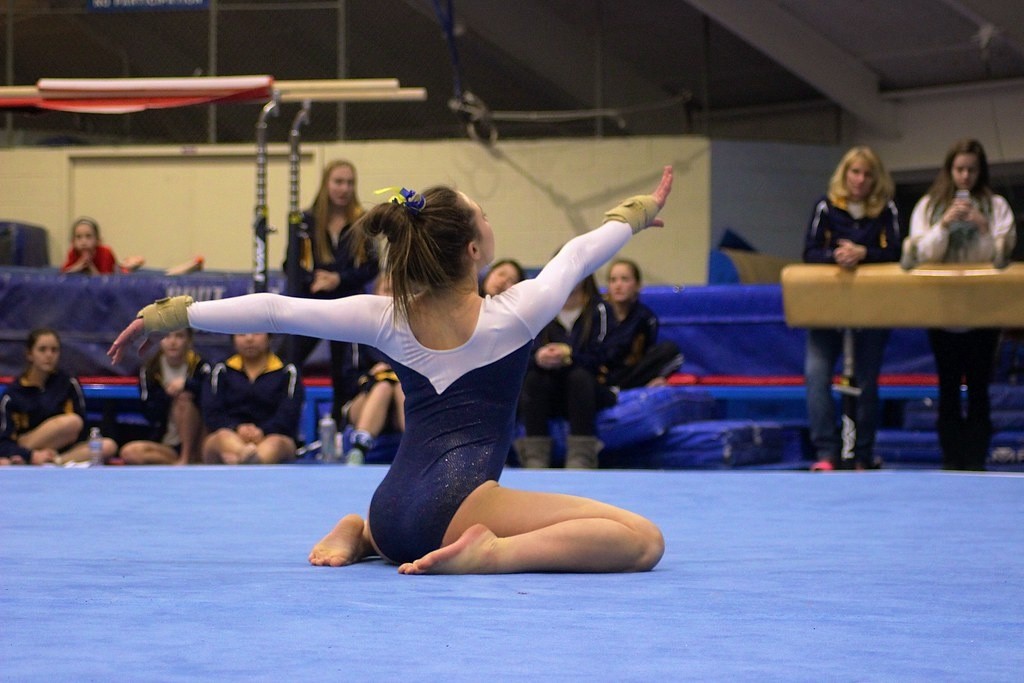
[89,427,103,467]
[318,412,338,464]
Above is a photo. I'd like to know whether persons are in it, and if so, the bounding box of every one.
[599,258,684,395]
[106,164,672,574]
[118,329,211,465]
[908,137,1017,472]
[803,147,907,471]
[0,329,118,467]
[476,259,526,299]
[511,247,614,465]
[273,160,382,389]
[330,275,406,465]
[60,219,204,278]
[199,332,304,465]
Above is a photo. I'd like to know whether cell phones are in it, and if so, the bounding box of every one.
[956,189,969,198]
